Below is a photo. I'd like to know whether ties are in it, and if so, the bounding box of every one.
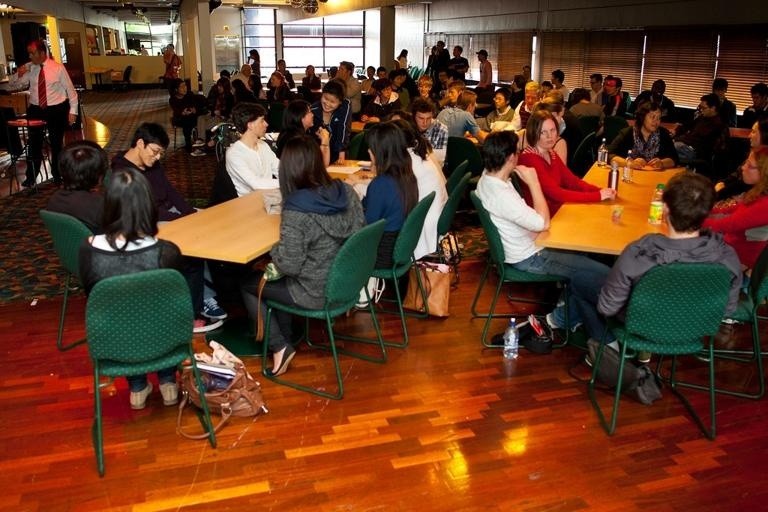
[38,63,47,110]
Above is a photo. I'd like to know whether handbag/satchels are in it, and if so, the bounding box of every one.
[251,257,286,281]
[174,348,269,440]
[402,259,451,318]
[587,336,663,406]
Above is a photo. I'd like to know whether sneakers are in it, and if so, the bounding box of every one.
[192,317,224,333]
[200,297,228,320]
[21,177,38,188]
[207,139,216,147]
[55,176,63,183]
[159,381,179,406]
[190,149,207,157]
[714,324,739,350]
[130,381,153,410]
[192,138,206,147]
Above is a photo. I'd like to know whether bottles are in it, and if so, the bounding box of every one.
[623,150,634,184]
[607,163,619,195]
[649,184,665,225]
[597,139,608,167]
[503,317,520,359]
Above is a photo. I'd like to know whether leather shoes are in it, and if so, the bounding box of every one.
[267,343,297,377]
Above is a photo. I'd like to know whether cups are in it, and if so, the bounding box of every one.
[610,205,624,223]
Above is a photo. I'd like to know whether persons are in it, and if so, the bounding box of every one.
[107,120,228,320]
[569,170,743,368]
[44,139,224,334]
[484,65,768,187]
[76,164,186,410]
[690,145,768,364]
[162,44,182,96]
[265,40,492,267]
[8,38,80,189]
[239,133,372,378]
[473,129,614,355]
[171,49,280,196]
